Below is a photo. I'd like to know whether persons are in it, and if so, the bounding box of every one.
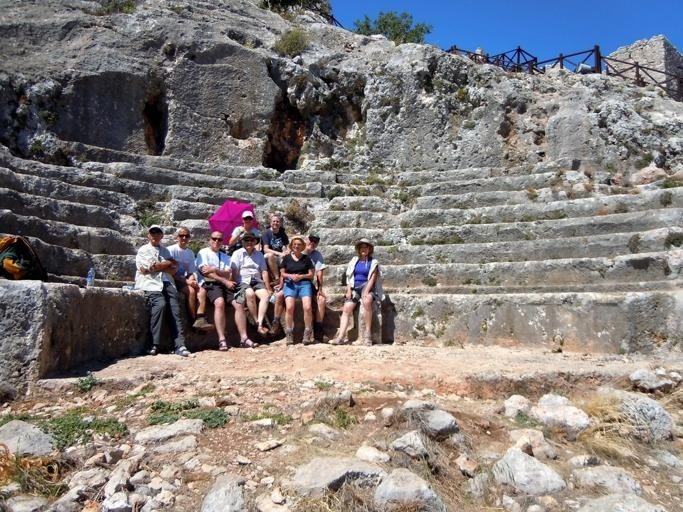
[327,238,378,345]
[134,210,289,357]
[279,239,313,344]
[304,234,330,343]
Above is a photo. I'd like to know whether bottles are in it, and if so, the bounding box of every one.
[86,267,95,287]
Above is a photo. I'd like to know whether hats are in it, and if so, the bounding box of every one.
[149,224,164,235]
[239,231,255,241]
[241,211,254,219]
[308,232,320,244]
[355,238,374,251]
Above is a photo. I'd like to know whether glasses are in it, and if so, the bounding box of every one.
[211,238,224,242]
[178,233,191,238]
[243,239,256,243]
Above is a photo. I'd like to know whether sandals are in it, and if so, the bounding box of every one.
[217,339,229,351]
[238,338,259,348]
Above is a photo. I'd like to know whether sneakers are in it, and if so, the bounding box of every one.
[302,327,313,345]
[268,323,281,336]
[364,337,373,347]
[286,328,295,345]
[145,344,160,356]
[190,317,215,335]
[254,321,269,337]
[173,345,191,356]
[328,336,346,346]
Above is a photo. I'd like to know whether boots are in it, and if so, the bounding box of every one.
[314,322,330,343]
